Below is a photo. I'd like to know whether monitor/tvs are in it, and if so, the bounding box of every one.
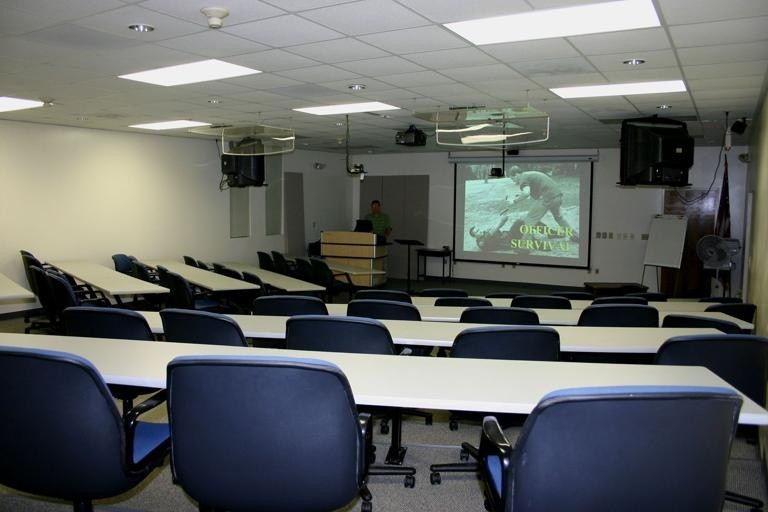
[354,219,372,232]
[221,142,266,187]
[619,117,693,184]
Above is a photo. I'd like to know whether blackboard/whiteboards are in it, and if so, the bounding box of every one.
[644,214,688,269]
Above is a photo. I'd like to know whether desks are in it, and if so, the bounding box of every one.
[134,310,727,354]
[321,243,390,288]
[0,332,567,416]
[356,265,387,276]
[0,273,41,315]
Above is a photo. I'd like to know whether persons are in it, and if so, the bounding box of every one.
[363,200,393,239]
[469,214,565,252]
[507,165,579,255]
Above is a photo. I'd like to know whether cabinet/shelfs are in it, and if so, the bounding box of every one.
[418,249,452,285]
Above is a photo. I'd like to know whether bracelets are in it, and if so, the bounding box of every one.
[513,199,517,204]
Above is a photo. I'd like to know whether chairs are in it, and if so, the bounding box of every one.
[653,335,765,511]
[20,249,113,333]
[253,297,329,316]
[161,309,246,346]
[183,256,270,297]
[62,308,156,343]
[430,386,743,512]
[166,354,417,511]
[0,345,166,510]
[436,326,560,430]
[286,316,432,435]
[347,287,759,333]
[256,250,355,298]
[113,254,220,310]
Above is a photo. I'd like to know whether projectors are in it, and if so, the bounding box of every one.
[396,129,427,146]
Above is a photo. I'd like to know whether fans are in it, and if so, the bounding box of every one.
[696,234,742,298]
